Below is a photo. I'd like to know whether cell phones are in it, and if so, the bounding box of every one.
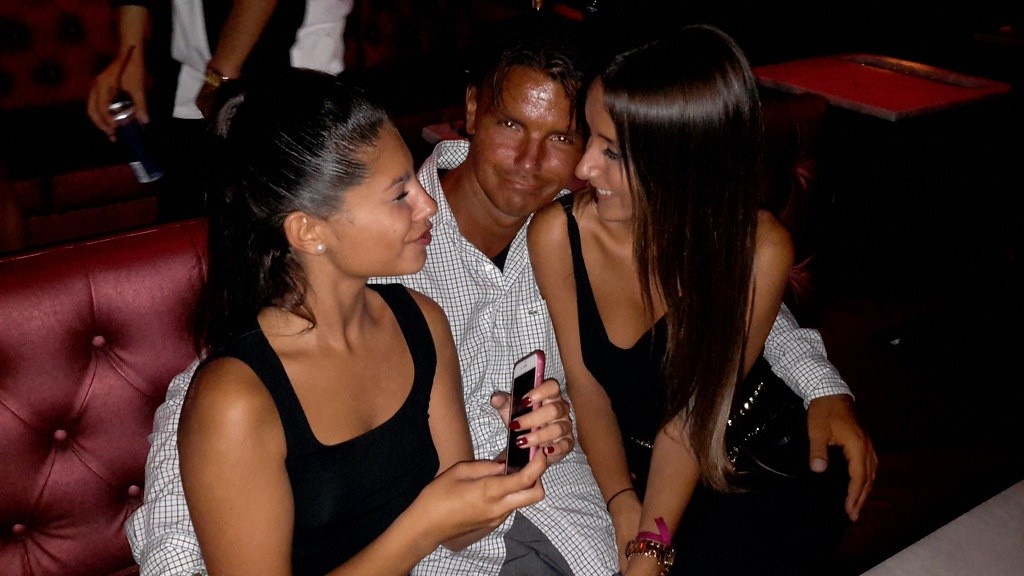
[503,350,545,474]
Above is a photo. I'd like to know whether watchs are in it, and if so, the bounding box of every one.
[626,540,676,570]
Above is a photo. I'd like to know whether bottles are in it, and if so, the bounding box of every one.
[109,92,172,196]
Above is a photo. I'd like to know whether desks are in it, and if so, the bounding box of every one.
[753,52,1012,122]
[858,480,1024,574]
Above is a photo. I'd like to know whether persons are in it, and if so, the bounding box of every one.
[196,0,354,211]
[124,1,882,576]
[177,70,574,576]
[87,1,277,225]
[525,25,797,575]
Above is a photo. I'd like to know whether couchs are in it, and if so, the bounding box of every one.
[0,197,207,575]
[2,1,389,105]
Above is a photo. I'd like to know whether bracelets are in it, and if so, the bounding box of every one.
[204,64,241,88]
[606,488,636,511]
[636,516,673,549]
